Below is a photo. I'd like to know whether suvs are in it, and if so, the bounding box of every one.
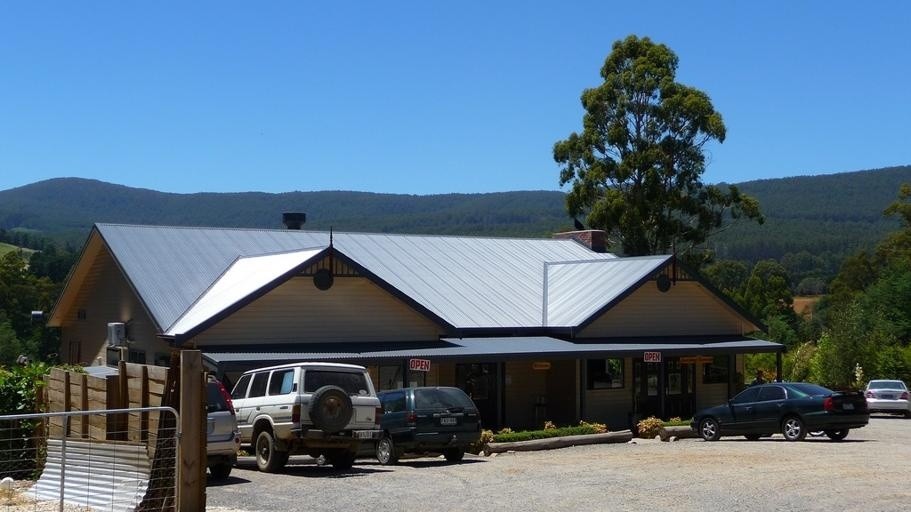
[168,371,242,486]
[226,361,385,474]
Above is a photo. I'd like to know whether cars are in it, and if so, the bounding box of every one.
[806,428,826,438]
[689,381,871,441]
[862,378,911,419]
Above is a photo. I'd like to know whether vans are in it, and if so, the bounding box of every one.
[315,386,481,463]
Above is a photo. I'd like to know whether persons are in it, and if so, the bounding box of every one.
[751,370,768,385]
